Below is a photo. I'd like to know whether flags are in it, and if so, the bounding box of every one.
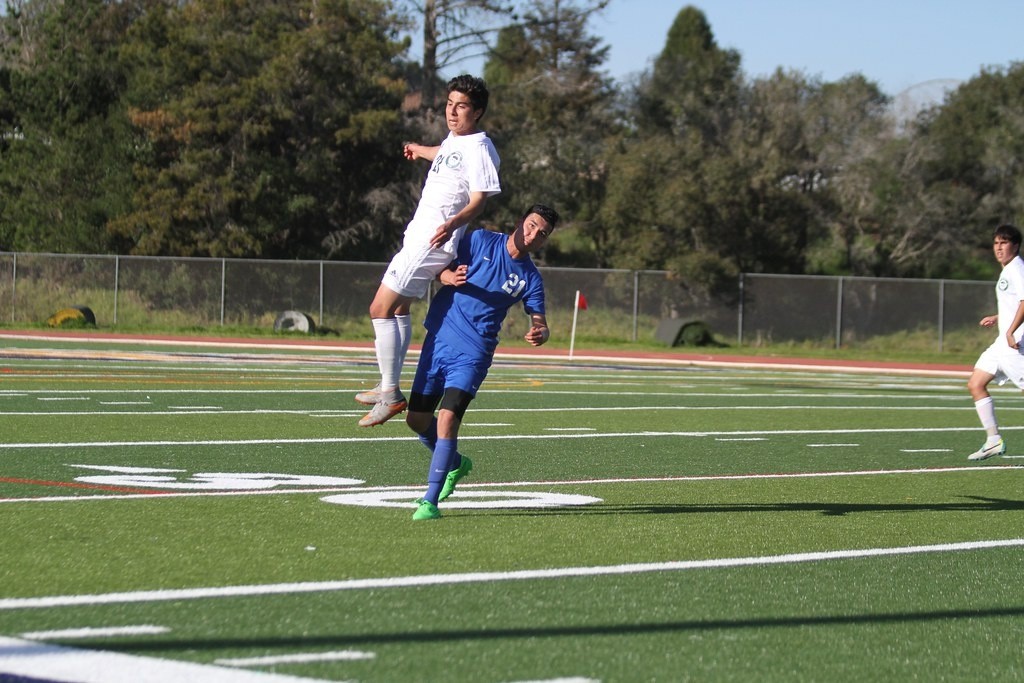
[577,294,587,308]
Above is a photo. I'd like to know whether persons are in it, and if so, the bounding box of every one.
[353,74,502,427]
[407,206,560,521]
[967,225,1024,460]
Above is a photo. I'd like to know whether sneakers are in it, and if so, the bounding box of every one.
[355,387,384,405]
[358,390,408,428]
[412,498,440,520]
[967,436,1006,461]
[438,456,472,502]
